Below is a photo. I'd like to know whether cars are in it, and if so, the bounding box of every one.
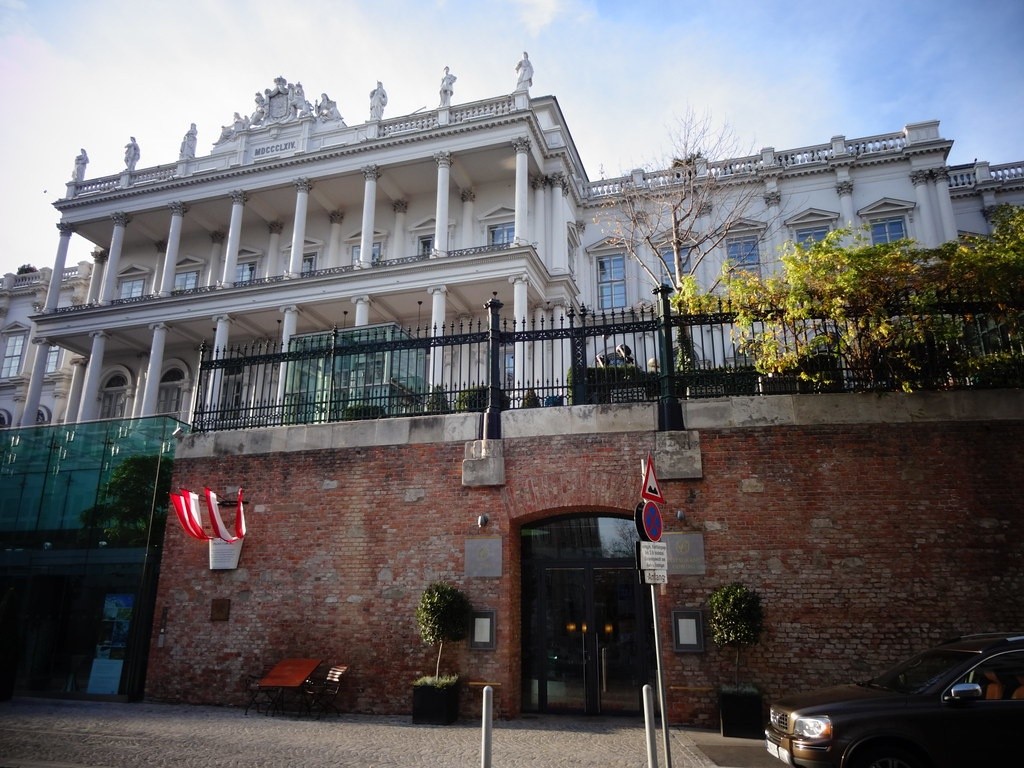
[763,632,1024,768]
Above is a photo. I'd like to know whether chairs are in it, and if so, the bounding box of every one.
[244,663,349,719]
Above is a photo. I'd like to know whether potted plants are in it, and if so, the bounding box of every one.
[708,583,765,739]
[412,582,475,725]
